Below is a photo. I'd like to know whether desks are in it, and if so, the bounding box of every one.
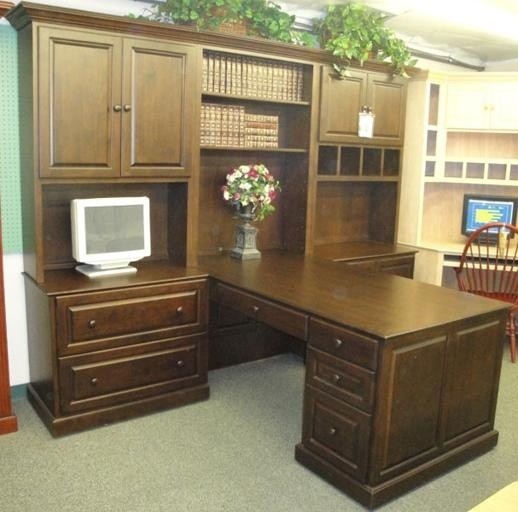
[398,241,518,286]
[200,249,513,512]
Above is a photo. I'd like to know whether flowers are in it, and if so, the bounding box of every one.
[219,164,281,214]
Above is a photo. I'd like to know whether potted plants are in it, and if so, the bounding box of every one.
[307,4,411,66]
[148,1,297,45]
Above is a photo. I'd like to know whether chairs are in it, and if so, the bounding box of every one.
[456,221,518,364]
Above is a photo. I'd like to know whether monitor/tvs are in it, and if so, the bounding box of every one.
[461,194,518,247]
[70,196,152,278]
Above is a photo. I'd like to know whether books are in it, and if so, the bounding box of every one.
[199,50,305,149]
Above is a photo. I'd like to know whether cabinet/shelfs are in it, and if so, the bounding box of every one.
[34,23,193,183]
[314,142,401,182]
[201,48,311,154]
[321,64,407,143]
[49,280,209,416]
[414,71,517,186]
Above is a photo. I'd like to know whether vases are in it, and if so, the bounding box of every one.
[231,210,262,262]
